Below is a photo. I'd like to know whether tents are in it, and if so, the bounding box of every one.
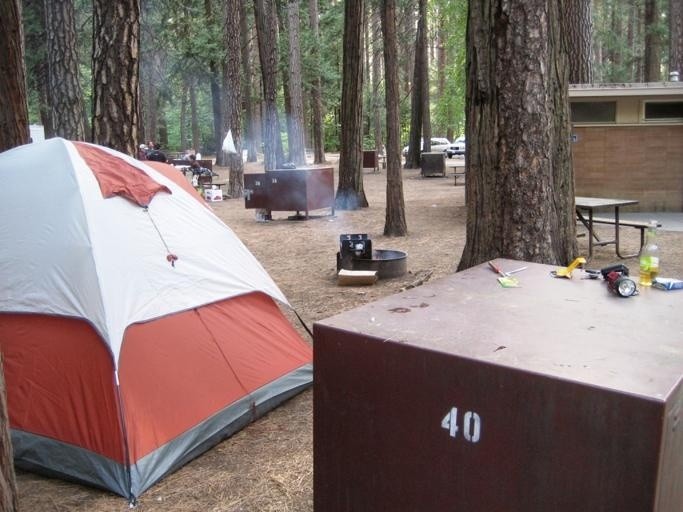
[2,136,315,502]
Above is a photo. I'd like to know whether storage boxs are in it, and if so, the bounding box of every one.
[204,189,223,202]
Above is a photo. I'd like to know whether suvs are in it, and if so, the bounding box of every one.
[445,136,465,158]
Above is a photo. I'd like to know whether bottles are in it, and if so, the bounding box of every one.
[637,220,660,286]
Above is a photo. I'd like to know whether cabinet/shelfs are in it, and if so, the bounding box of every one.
[242,168,335,219]
[420,152,446,178]
[362,151,380,174]
[172,159,213,182]
[311,258,683,511]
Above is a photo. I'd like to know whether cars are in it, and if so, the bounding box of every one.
[400,136,453,160]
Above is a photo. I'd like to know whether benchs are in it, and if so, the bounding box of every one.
[447,172,465,185]
[576,216,662,256]
[200,183,226,190]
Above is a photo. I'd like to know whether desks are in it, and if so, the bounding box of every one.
[575,194,639,260]
[197,174,218,190]
[446,162,465,185]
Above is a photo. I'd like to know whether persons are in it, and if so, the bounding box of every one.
[137,142,213,197]
